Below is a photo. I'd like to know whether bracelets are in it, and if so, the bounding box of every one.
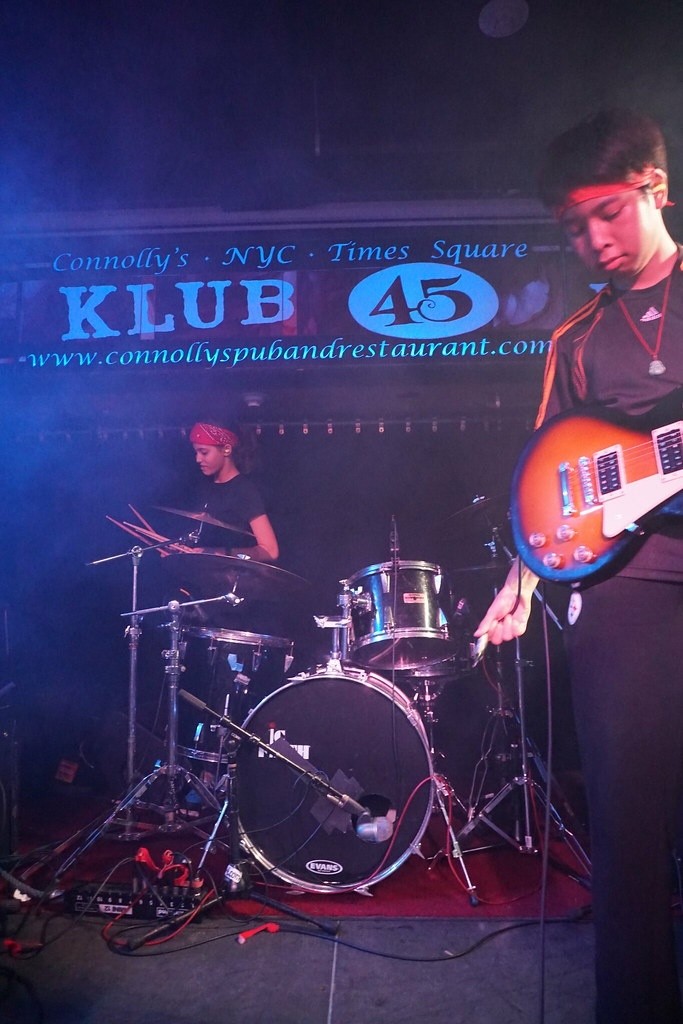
[224,548,232,556]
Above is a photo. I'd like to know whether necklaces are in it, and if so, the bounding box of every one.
[618,272,674,376]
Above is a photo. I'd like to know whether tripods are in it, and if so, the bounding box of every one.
[20,510,591,950]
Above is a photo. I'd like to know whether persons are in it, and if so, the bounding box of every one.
[160,418,279,803]
[473,104,683,1023]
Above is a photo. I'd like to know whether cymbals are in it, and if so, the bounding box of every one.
[147,505,257,539]
[451,560,511,576]
[438,491,510,526]
[163,552,313,593]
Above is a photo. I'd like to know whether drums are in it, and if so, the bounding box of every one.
[312,614,351,668]
[347,560,460,670]
[176,626,293,763]
[236,667,434,892]
[396,615,469,679]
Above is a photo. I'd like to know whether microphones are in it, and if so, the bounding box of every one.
[357,816,393,844]
[388,514,399,558]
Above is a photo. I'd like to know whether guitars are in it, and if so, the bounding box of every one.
[511,387,682,591]
